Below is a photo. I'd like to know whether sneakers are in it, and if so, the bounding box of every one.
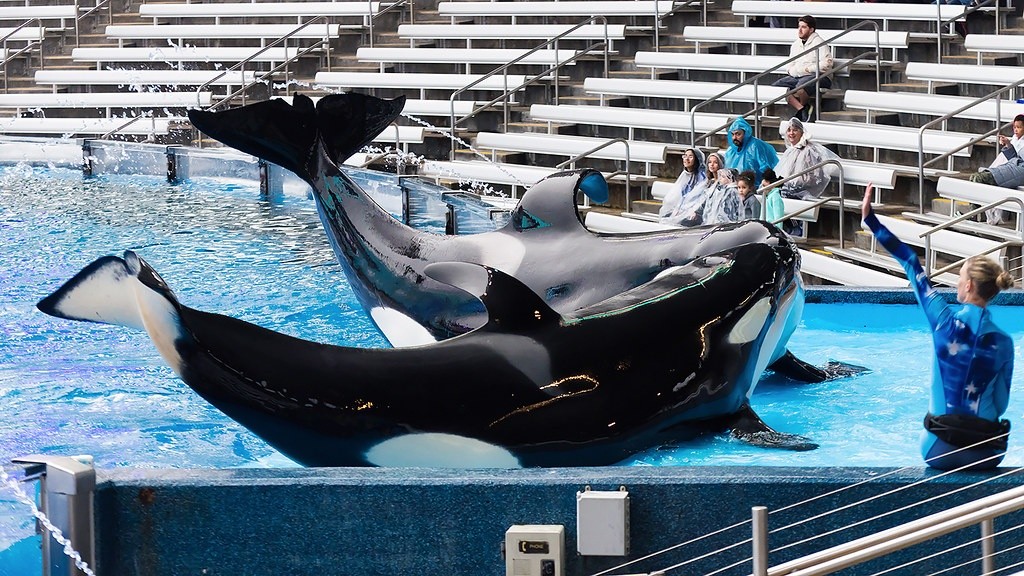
[801,104,814,122]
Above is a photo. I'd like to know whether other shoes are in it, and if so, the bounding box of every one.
[956,210,971,220]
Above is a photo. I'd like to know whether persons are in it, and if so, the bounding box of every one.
[954,114,1024,224]
[860,180,1017,472]
[657,115,842,231]
[771,15,834,123]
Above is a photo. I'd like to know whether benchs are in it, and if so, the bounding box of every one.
[0,0,1024,286]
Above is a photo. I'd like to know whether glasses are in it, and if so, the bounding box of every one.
[682,154,693,160]
